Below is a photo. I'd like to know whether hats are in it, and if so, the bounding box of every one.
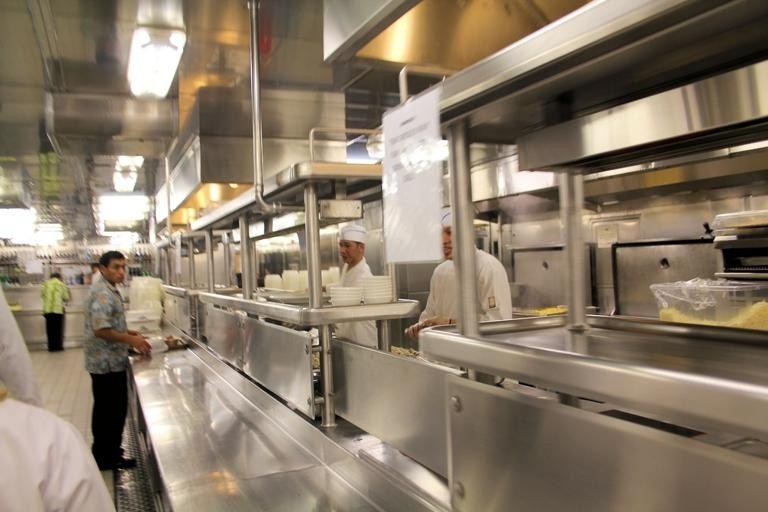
[340,224,366,244]
[442,205,451,226]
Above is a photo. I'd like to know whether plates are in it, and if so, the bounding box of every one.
[330,275,392,306]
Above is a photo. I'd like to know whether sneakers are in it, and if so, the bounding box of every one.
[100,455,136,471]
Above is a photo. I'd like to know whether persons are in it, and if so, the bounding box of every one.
[39,273,70,352]
[91,263,102,284]
[402,207,520,387]
[319,225,379,350]
[0,377,117,512]
[81,251,153,470]
